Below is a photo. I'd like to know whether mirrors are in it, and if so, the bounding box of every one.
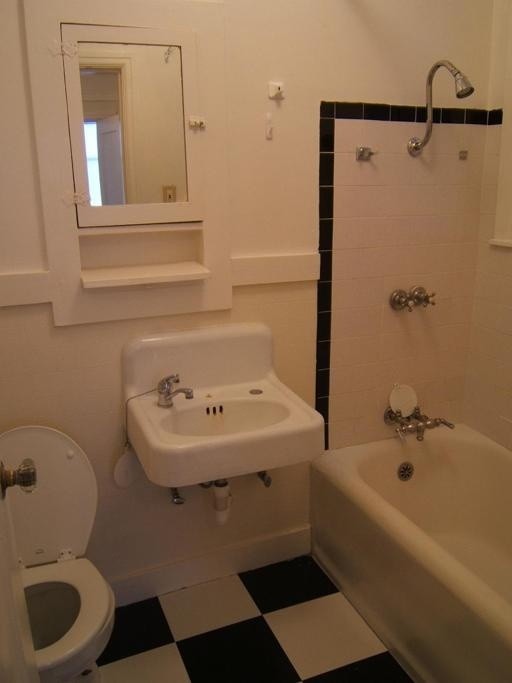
[61,23,206,225]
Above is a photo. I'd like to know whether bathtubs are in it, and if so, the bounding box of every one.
[310,423,512,681]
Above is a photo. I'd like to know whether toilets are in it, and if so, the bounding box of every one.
[1,426,116,683]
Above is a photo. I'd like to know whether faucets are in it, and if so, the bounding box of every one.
[156,374,194,407]
[391,407,456,442]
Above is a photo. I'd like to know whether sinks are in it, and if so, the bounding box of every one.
[122,321,324,489]
